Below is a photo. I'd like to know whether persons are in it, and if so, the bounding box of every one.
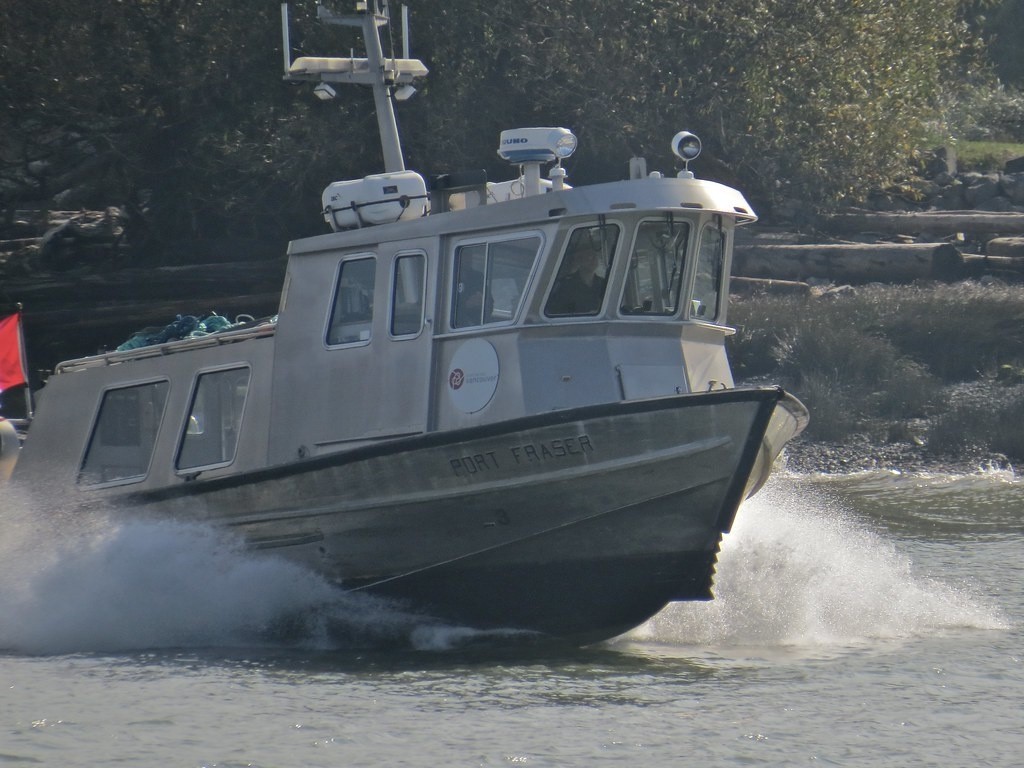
[546,228,607,317]
[451,254,494,328]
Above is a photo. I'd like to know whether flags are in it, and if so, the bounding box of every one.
[2,314,25,394]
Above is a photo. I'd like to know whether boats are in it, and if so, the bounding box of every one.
[0,0,814,663]
